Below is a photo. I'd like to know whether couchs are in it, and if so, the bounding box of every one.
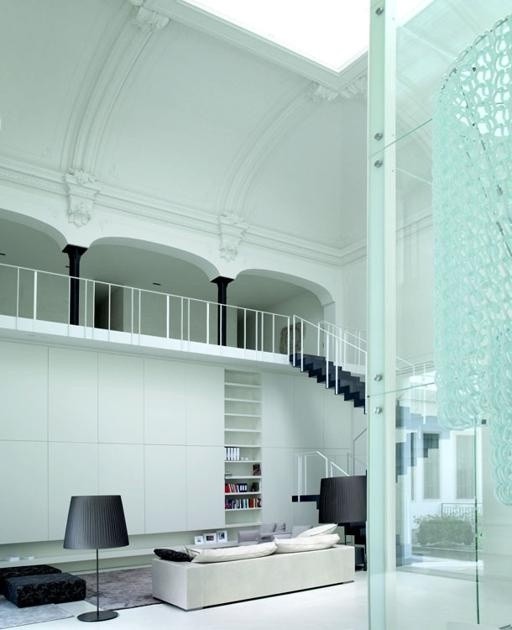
[152,544,356,612]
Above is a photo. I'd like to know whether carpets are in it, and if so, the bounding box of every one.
[72,565,162,612]
[0,595,72,630]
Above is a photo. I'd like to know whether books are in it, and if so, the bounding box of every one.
[223,447,261,510]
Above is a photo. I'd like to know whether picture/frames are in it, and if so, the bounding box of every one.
[193,531,227,544]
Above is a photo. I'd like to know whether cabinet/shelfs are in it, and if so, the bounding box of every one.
[223,369,264,529]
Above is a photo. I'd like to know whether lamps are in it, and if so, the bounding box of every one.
[317,476,366,545]
[60,497,131,624]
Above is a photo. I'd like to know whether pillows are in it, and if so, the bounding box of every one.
[297,521,339,536]
[152,547,191,563]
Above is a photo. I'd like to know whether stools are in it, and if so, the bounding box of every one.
[0,565,88,608]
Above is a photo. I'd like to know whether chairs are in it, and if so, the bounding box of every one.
[238,521,313,543]
